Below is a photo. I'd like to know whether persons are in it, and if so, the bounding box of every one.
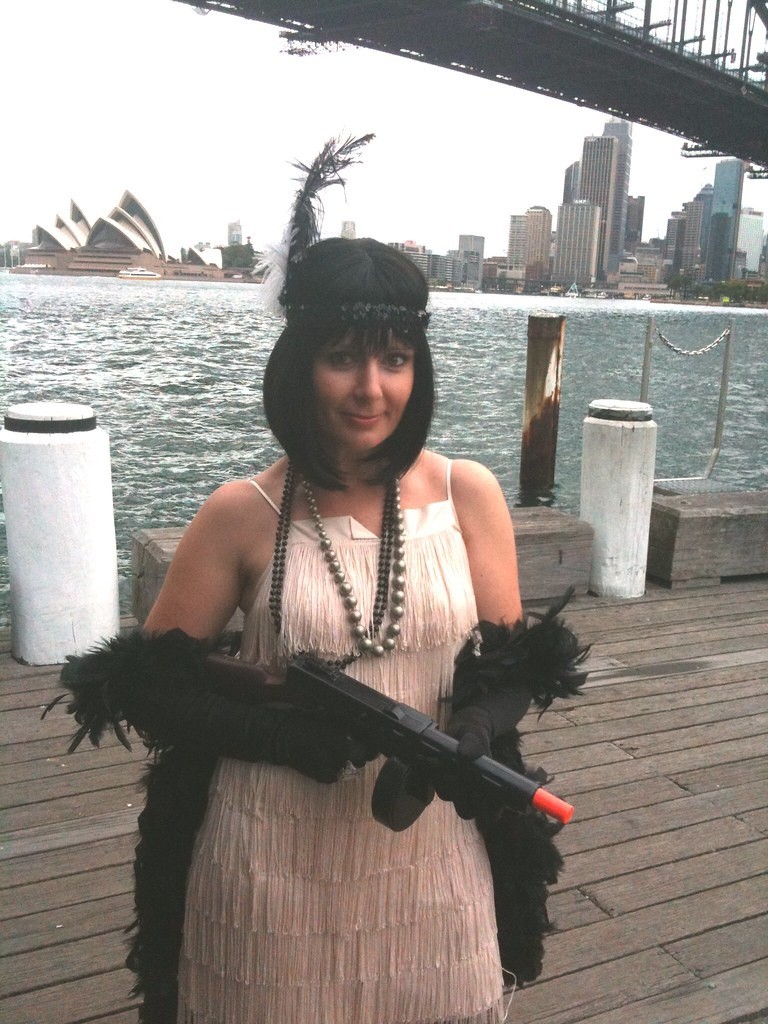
[132,236,531,1024]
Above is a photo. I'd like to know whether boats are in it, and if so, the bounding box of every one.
[119,266,162,281]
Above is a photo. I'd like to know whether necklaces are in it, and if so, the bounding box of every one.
[270,451,407,666]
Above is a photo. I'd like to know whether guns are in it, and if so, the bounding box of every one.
[286,651,575,832]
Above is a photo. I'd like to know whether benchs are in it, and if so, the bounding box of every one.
[647,490,768,589]
[132,507,596,633]
